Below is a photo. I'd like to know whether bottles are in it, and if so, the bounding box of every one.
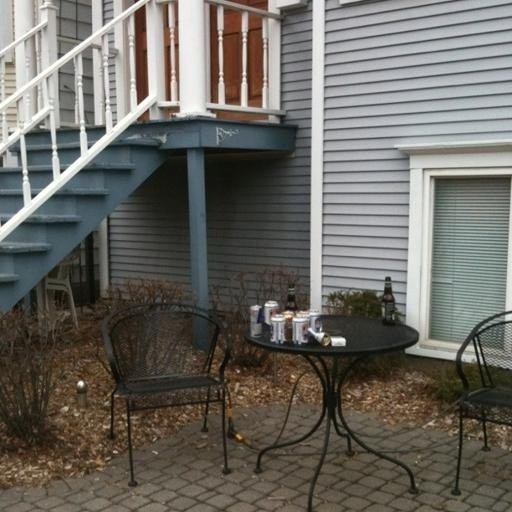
[380,275,397,326]
[286,283,298,312]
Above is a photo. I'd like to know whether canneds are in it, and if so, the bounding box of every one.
[270,310,331,348]
[263,300,279,326]
[248,304,263,339]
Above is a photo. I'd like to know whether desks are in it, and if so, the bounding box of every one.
[246,312,420,512]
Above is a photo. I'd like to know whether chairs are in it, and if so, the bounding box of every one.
[451,310,511,495]
[101,300,234,488]
